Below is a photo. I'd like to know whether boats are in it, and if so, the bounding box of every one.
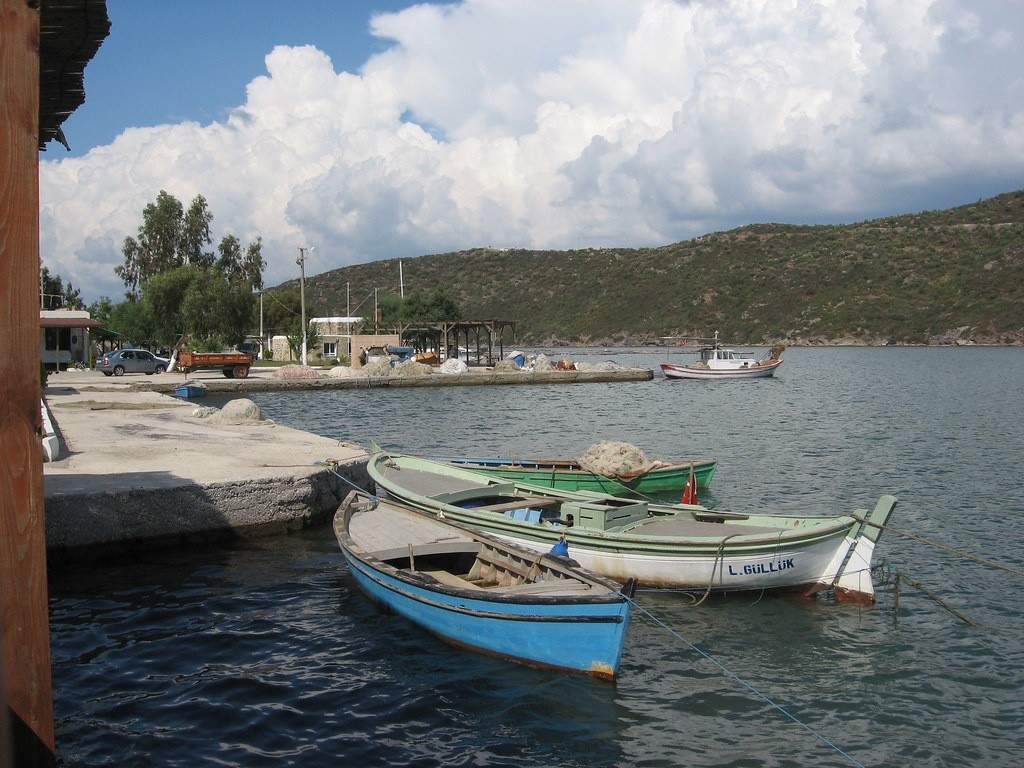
[366,440,900,608]
[660,329,785,379]
[404,456,719,500]
[332,489,640,685]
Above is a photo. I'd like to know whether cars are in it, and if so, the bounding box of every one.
[95,348,172,377]
